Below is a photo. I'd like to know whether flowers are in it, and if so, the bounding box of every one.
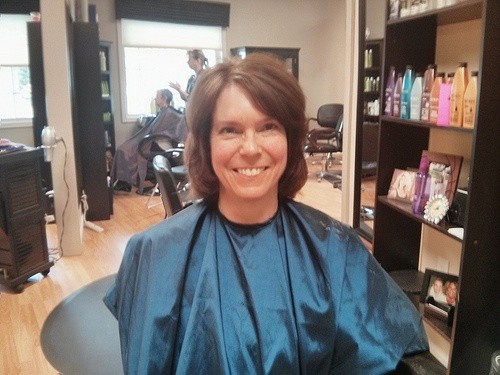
[424,195,449,223]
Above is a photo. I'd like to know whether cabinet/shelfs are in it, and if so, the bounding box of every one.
[373,0,500,375]
[100,39,116,176]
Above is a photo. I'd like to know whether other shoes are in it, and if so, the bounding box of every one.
[115,183,132,192]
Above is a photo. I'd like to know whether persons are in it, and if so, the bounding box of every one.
[110,89,183,192]
[103,54,430,375]
[169,49,211,105]
[388,174,409,200]
[427,277,458,307]
[434,166,451,194]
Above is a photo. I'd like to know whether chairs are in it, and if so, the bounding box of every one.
[136,132,205,219]
[302,104,343,181]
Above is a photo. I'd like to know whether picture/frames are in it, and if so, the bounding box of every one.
[413,150,463,219]
[420,267,459,314]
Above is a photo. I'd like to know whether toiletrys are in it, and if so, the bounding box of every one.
[420,63,436,122]
[449,62,469,128]
[446,71,455,84]
[393,72,403,117]
[410,72,424,121]
[428,71,446,124]
[437,83,451,128]
[462,70,478,129]
[400,64,415,120]
[384,65,396,116]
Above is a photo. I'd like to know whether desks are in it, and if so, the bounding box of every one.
[0,142,55,292]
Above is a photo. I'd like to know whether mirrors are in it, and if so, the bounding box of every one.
[353,38,384,243]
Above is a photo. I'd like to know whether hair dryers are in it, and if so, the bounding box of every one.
[41,126,63,162]
